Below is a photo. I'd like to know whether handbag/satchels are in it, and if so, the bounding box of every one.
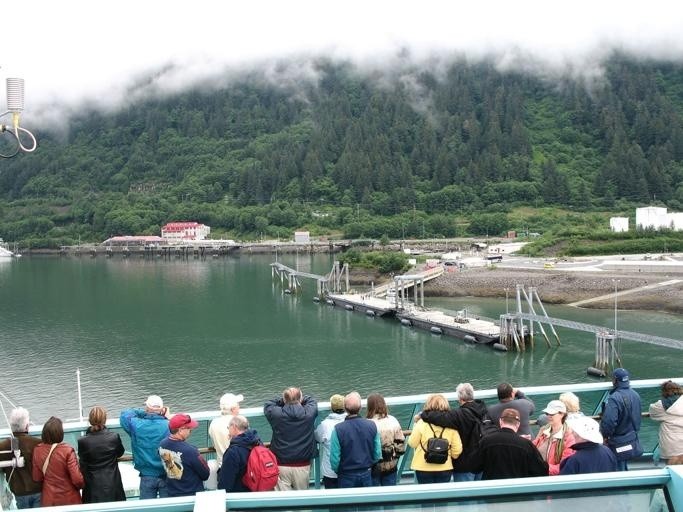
[605,392,643,463]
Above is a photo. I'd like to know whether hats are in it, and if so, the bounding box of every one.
[541,399,569,418]
[330,392,348,413]
[563,412,604,446]
[143,393,165,411]
[498,407,522,422]
[610,367,632,391]
[169,413,200,432]
[219,393,245,405]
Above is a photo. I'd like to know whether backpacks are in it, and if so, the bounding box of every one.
[419,421,452,464]
[231,440,280,492]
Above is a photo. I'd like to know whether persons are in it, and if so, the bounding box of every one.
[330,390,383,512]
[157,413,210,498]
[649,380,683,465]
[314,393,348,512]
[407,394,463,511]
[558,416,617,501]
[532,399,576,475]
[208,392,244,466]
[120,395,171,500]
[216,416,279,494]
[0,407,43,509]
[470,408,549,505]
[263,386,319,512]
[31,416,84,507]
[413,383,489,511]
[599,368,643,471]
[486,382,536,442]
[559,391,584,439]
[78,406,127,504]
[365,391,407,512]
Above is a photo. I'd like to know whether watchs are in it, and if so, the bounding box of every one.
[418,411,423,418]
